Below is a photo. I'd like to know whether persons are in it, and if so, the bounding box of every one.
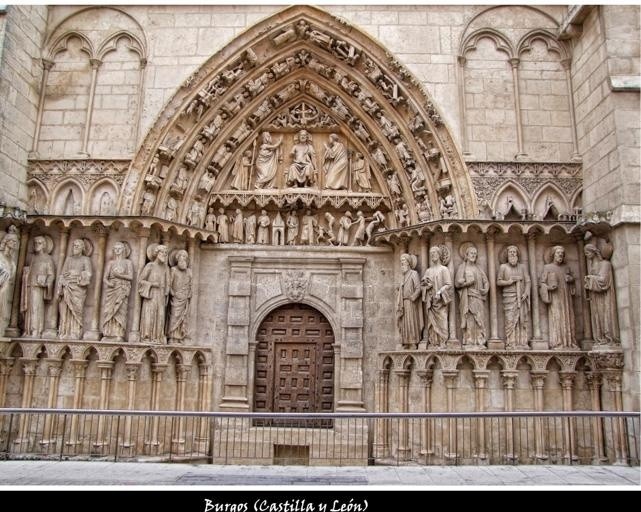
[167,250,194,345]
[99,240,135,343]
[56,239,93,340]
[139,21,459,245]
[497,246,532,351]
[21,236,56,338]
[0,234,21,337]
[395,253,422,350]
[454,242,489,349]
[138,245,171,343]
[420,247,452,349]
[583,244,622,348]
[539,245,581,350]
